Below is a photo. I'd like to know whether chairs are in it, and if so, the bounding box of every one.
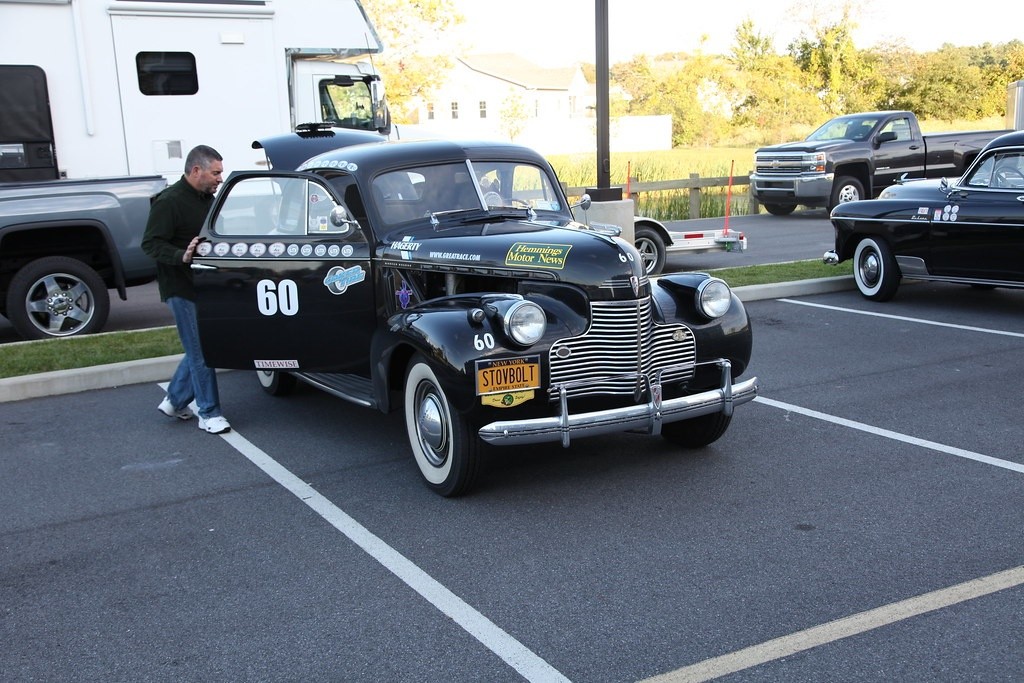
[851,124,872,140]
[307,173,492,232]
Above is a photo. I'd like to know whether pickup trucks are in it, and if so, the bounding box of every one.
[748,111,1018,219]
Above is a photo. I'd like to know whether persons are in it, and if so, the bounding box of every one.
[141,145,232,434]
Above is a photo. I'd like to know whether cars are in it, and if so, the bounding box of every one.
[822,130,1024,308]
[184,119,767,503]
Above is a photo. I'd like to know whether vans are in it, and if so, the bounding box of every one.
[0,0,408,342]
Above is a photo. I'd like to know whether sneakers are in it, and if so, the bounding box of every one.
[199,410,231,433]
[158,394,194,420]
